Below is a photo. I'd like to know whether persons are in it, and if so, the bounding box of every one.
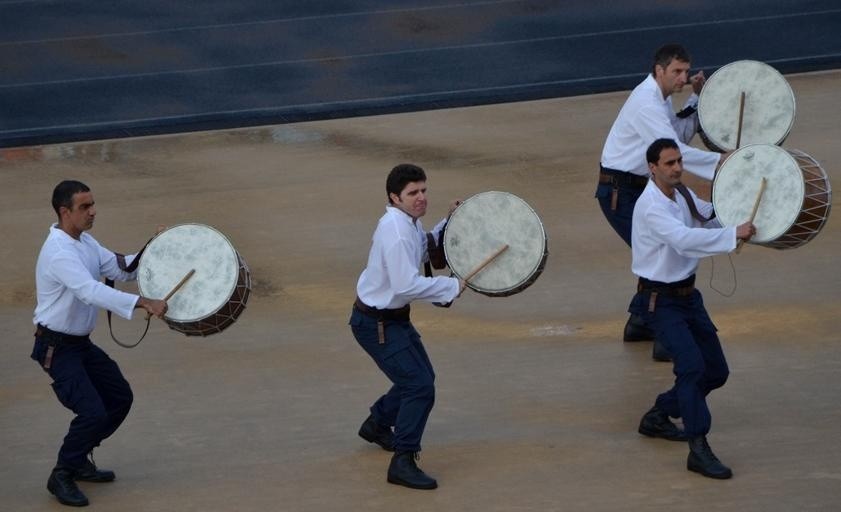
[594,41,734,362]
[29,180,169,507]
[347,164,467,490]
[630,138,756,479]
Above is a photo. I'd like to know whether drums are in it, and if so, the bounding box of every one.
[138,224,251,337]
[443,190,548,297]
[713,142,831,250]
[696,61,795,155]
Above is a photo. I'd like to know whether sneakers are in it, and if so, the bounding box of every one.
[638,406,688,442]
[358,415,396,451]
[387,452,438,490]
[652,336,678,361]
[687,434,733,479]
[47,477,90,507]
[623,313,655,342]
[72,458,115,482]
